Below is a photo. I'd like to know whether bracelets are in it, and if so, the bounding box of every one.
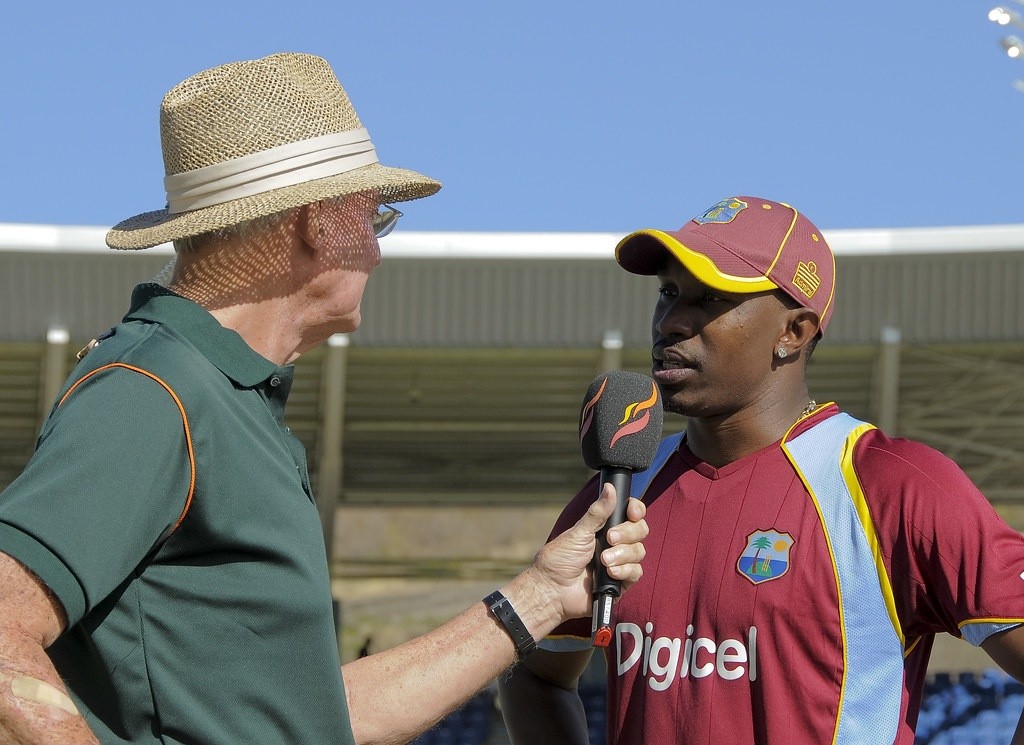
[482,590,538,660]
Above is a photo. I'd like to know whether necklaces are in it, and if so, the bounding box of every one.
[795,399,816,423]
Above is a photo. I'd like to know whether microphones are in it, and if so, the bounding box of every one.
[579,369,664,647]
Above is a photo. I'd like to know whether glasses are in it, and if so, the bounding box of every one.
[356,190,403,239]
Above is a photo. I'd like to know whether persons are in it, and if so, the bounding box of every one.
[0,51,649,745]
[496,195,1024,745]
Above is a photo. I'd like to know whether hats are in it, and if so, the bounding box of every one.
[104,53,442,252]
[615,195,835,339]
